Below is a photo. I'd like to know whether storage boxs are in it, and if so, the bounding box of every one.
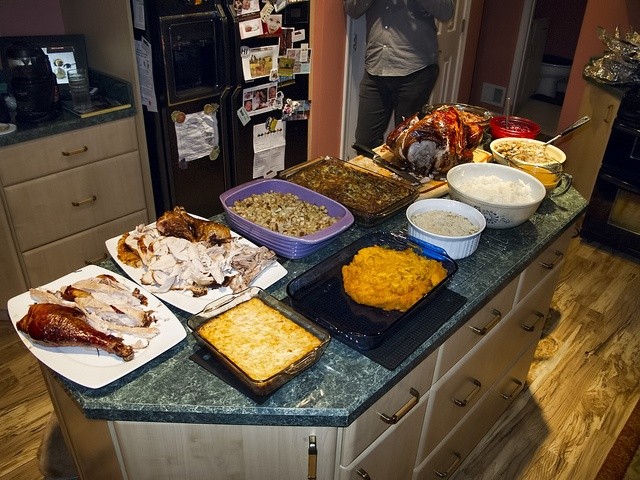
[607,188,640,236]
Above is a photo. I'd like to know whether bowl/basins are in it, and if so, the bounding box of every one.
[446,162,547,229]
[422,102,494,134]
[488,115,542,139]
[405,197,486,260]
[490,137,567,168]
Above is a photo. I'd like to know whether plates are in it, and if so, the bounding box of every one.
[104,210,289,320]
[0,122,17,135]
[7,264,188,390]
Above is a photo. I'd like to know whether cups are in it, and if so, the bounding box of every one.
[66,68,95,114]
[508,152,574,203]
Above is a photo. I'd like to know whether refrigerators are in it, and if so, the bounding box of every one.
[131,0,311,219]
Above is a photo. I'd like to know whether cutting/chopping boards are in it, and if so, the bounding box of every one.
[343,142,494,203]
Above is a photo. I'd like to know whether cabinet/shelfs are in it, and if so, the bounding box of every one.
[512,15,552,116]
[339,214,587,480]
[1,114,157,319]
[563,83,622,232]
[338,0,396,161]
[37,364,337,480]
[578,86,640,258]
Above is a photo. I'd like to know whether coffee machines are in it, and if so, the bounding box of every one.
[3,39,62,123]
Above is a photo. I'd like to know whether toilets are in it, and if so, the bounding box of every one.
[536,55,573,96]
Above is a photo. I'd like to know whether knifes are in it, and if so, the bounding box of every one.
[351,141,424,187]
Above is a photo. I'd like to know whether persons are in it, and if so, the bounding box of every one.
[253,90,260,109]
[259,90,267,102]
[269,86,277,98]
[244,100,252,112]
[262,15,282,34]
[344,0,456,156]
